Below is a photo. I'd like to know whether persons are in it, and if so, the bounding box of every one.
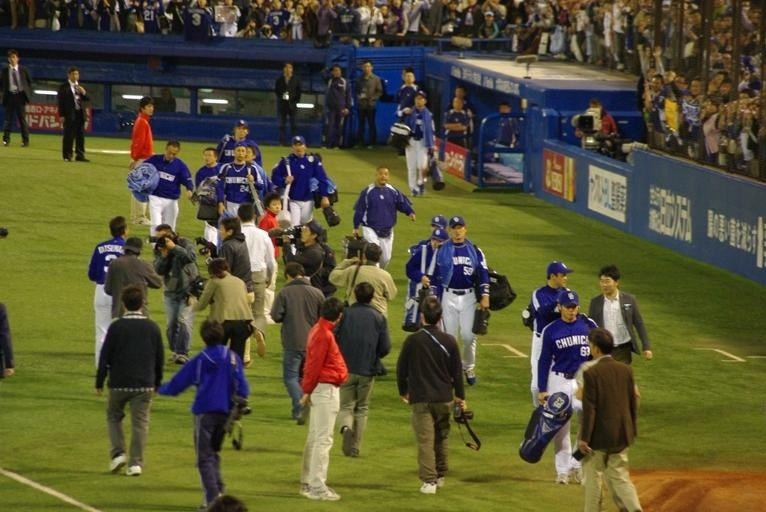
[526,1,765,183]
[0,301,15,379]
[88,118,490,510]
[522,261,653,510]
[1,0,525,197]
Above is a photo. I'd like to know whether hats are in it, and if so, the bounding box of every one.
[547,262,574,274]
[291,135,306,145]
[431,215,465,240]
[233,141,248,147]
[234,120,249,127]
[560,291,580,308]
[121,236,144,255]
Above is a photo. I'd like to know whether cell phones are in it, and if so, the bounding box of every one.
[572,448,587,461]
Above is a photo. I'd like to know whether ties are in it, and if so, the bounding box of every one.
[12,69,19,95]
[74,84,81,108]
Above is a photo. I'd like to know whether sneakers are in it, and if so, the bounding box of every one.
[437,477,446,488]
[467,370,476,385]
[420,483,437,494]
[300,483,341,501]
[571,467,584,483]
[110,454,127,474]
[351,448,360,457]
[128,466,143,477]
[557,473,568,485]
[343,428,353,456]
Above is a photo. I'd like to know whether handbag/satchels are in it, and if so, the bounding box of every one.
[428,155,444,190]
[474,270,516,311]
[276,195,292,227]
[519,392,573,464]
[310,175,337,208]
[402,274,439,332]
[387,123,411,150]
[371,225,392,238]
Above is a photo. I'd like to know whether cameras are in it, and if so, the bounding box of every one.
[454,405,472,424]
[235,396,251,414]
[156,235,171,246]
